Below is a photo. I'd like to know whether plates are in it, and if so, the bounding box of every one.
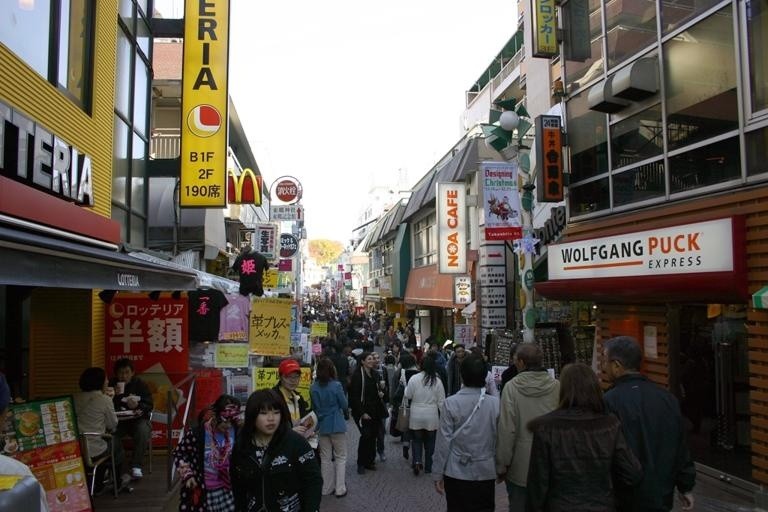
[4,442,19,453]
[55,493,69,504]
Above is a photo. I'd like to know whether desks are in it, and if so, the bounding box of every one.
[111,406,145,491]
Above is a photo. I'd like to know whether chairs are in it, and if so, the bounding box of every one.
[139,398,157,474]
[79,433,119,499]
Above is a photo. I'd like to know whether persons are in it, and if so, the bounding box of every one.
[71,366,134,497]
[0,453,49,512]
[309,358,349,498]
[429,353,502,511]
[229,388,324,511]
[169,392,243,511]
[290,292,417,461]
[348,335,522,495]
[523,360,645,511]
[493,341,561,510]
[106,357,155,478]
[599,335,698,511]
[271,359,321,471]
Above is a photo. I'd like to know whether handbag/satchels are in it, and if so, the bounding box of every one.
[393,381,404,403]
[396,406,410,432]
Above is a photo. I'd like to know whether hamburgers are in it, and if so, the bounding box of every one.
[19,412,40,434]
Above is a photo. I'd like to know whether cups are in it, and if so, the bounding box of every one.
[117,382,125,394]
[67,473,73,483]
[75,472,81,480]
[5,436,10,444]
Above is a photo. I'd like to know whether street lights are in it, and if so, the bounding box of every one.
[480,94,541,343]
[291,224,308,299]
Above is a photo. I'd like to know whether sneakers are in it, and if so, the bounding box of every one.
[132,468,143,477]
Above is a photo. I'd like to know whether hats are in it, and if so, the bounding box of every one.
[279,360,301,374]
